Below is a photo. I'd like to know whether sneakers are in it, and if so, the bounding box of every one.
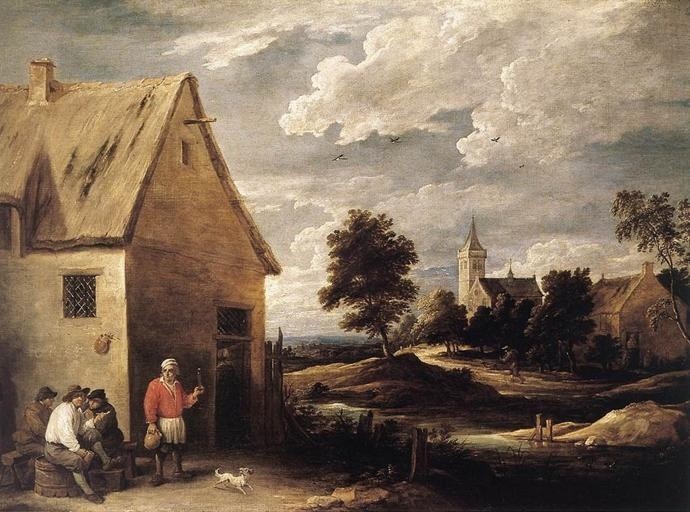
[174,471,193,479]
[102,456,125,471]
[86,491,104,504]
[149,474,164,486]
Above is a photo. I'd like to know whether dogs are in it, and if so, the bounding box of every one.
[213,467,253,495]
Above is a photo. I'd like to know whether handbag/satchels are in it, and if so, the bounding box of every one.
[144,432,163,450]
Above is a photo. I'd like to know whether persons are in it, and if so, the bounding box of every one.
[12,385,58,456]
[143,357,205,486]
[499,345,527,384]
[80,388,125,458]
[43,383,125,505]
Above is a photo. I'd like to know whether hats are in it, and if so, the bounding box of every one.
[34,386,57,400]
[87,389,105,399]
[62,385,90,402]
[161,358,178,369]
